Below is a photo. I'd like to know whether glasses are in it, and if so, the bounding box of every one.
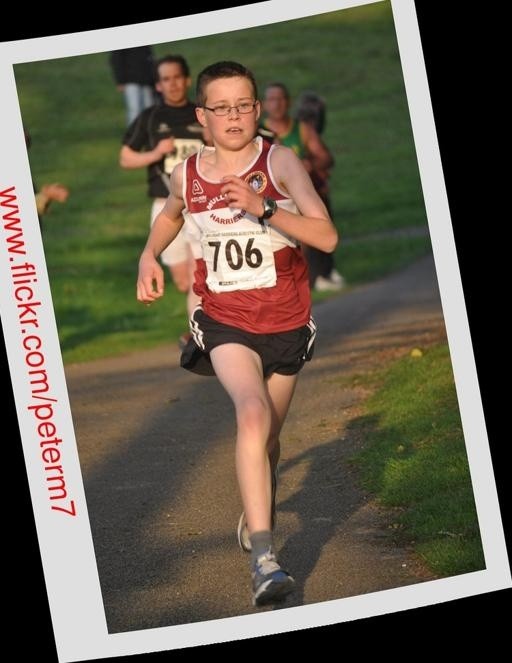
[203,99,257,116]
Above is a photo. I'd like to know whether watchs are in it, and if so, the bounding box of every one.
[258,195,278,219]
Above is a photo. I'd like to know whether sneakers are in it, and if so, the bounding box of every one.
[250,553,296,607]
[237,471,277,553]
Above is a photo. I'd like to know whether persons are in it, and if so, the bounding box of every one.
[24,125,70,217]
[296,93,345,293]
[118,53,215,293]
[136,58,340,607]
[258,81,333,175]
[110,45,162,127]
